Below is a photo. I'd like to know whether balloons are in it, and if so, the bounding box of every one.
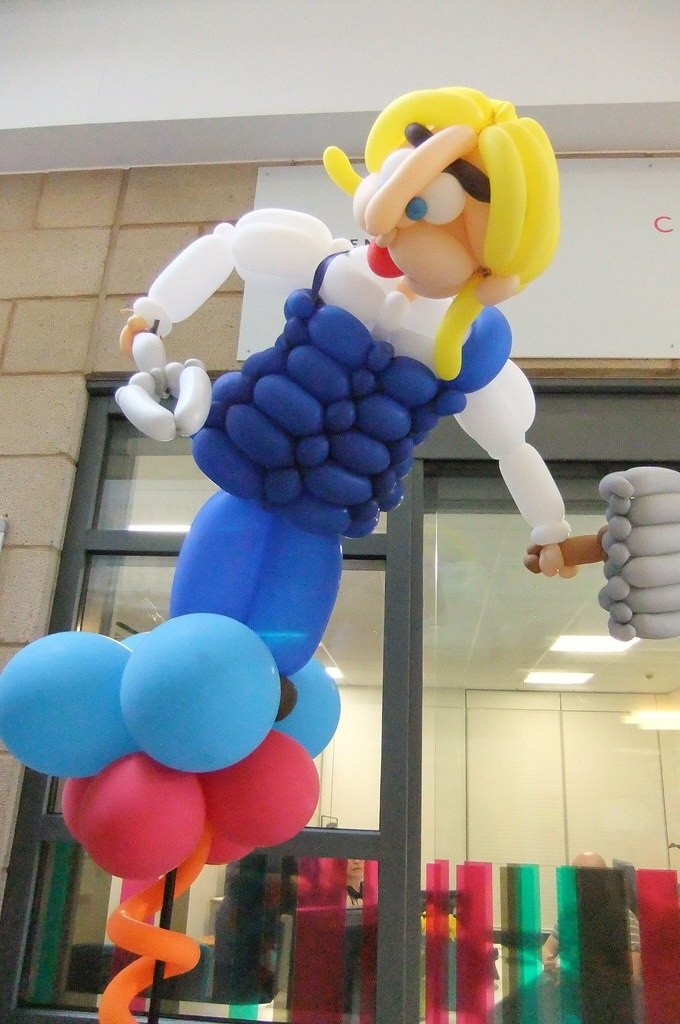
[119,613,281,773]
[197,729,319,848]
[62,778,93,838]
[205,825,252,866]
[1,628,139,779]
[80,752,206,882]
[120,631,150,652]
[525,466,679,642]
[273,657,342,758]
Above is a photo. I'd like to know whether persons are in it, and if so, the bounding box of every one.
[347,859,363,904]
[112,85,569,725]
[216,850,322,1003]
[540,852,644,1024]
